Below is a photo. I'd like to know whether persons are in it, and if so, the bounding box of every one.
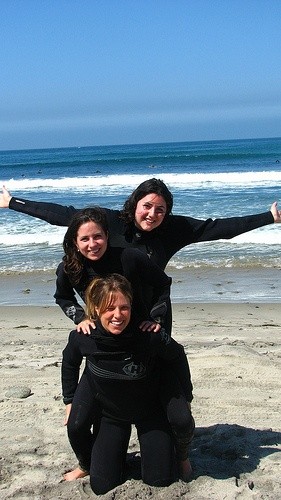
[0,178,281,338]
[61,273,194,496]
[55,205,196,482]
